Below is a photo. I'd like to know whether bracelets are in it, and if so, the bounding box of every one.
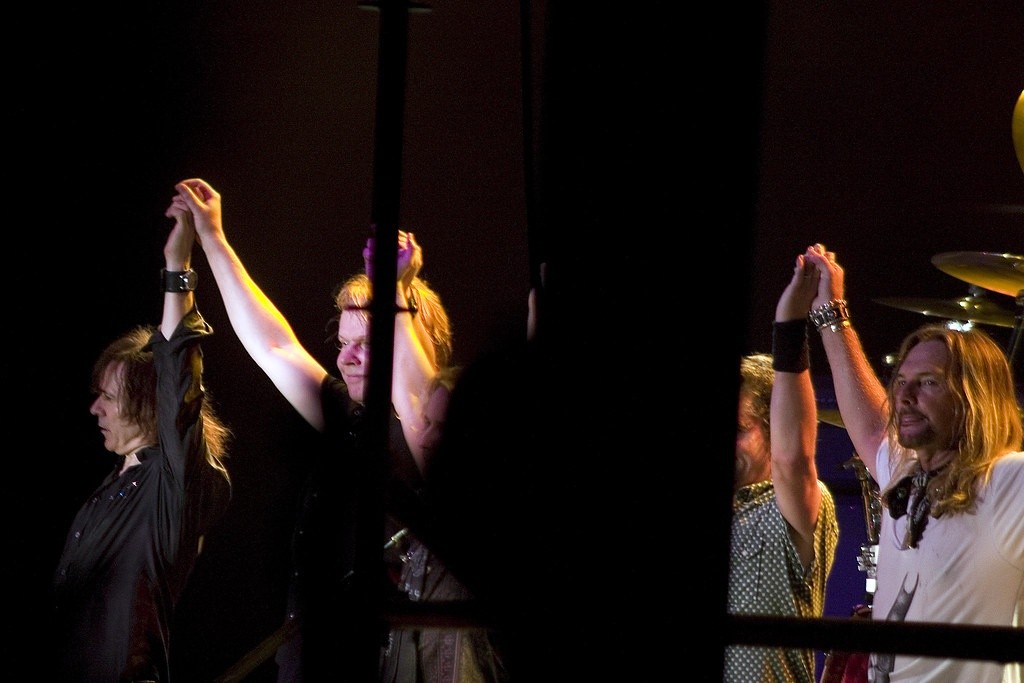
[808,299,851,332]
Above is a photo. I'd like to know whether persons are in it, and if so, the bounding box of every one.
[54,178,822,683]
[808,246,1024,683]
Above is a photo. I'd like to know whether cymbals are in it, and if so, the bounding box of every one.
[816,398,847,429]
[869,291,1024,329]
[931,250,1024,297]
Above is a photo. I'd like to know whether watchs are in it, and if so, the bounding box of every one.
[160,268,198,293]
